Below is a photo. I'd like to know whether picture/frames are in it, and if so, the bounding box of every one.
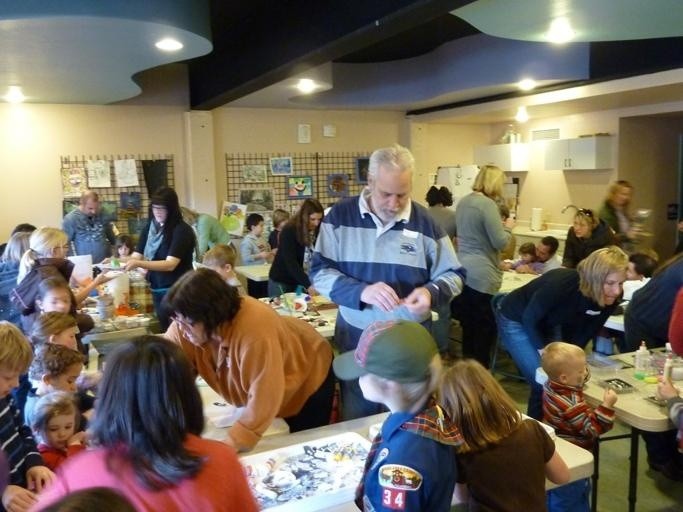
[286,175,314,200]
[269,157,293,176]
[355,156,371,185]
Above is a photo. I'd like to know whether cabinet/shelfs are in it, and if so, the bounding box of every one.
[474,135,614,172]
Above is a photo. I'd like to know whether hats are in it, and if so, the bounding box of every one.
[330,318,440,385]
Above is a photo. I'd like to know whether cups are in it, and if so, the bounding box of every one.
[497,131,521,145]
[97,296,148,329]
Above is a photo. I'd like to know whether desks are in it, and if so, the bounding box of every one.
[60,255,682,511]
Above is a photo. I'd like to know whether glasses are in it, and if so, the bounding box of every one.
[167,314,206,331]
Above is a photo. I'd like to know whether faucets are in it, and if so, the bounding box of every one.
[561,204,579,214]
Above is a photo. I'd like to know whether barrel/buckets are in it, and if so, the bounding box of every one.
[64,254,93,282]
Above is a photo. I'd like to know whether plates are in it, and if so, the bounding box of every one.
[278,291,311,319]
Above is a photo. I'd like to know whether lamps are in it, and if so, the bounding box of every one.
[513,104,530,124]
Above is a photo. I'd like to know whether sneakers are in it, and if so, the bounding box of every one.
[647,452,683,485]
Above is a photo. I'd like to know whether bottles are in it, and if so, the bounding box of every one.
[663,353,674,381]
[635,340,650,372]
